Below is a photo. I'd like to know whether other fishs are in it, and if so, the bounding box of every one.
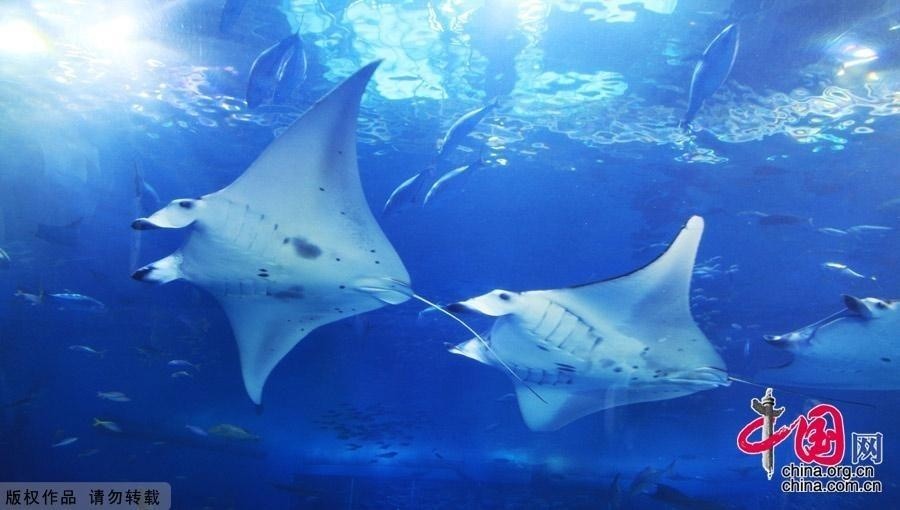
[0,15,896,510]
